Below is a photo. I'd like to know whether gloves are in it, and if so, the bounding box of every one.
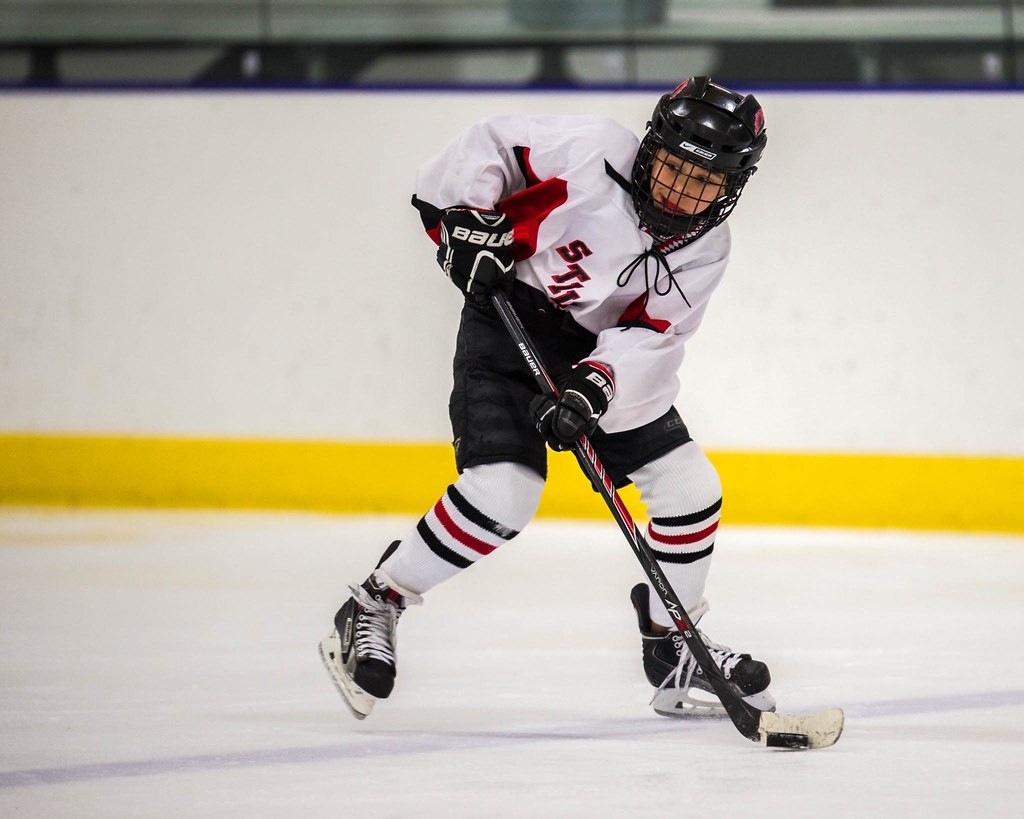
[525,362,616,451]
[437,207,517,298]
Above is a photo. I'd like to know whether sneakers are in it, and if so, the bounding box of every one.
[319,540,425,721]
[630,583,775,718]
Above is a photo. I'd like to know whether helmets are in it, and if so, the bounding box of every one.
[631,76,767,243]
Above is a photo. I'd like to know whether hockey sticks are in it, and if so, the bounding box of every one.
[490,290,846,752]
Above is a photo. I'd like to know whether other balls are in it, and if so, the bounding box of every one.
[765,732,809,750]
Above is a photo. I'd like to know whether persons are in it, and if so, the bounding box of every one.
[317,74,778,721]
